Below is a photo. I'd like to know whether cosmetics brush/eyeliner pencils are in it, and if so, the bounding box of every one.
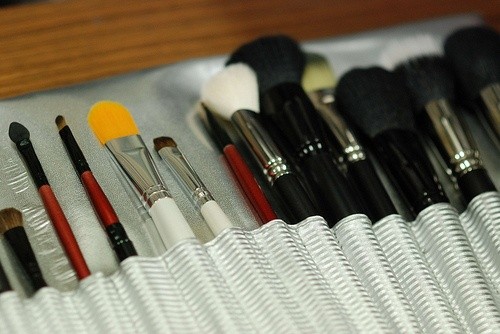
[197,22,500,227]
[89,99,199,255]
[152,134,233,240]
[0,206,79,332]
[7,120,94,283]
[54,113,139,265]
